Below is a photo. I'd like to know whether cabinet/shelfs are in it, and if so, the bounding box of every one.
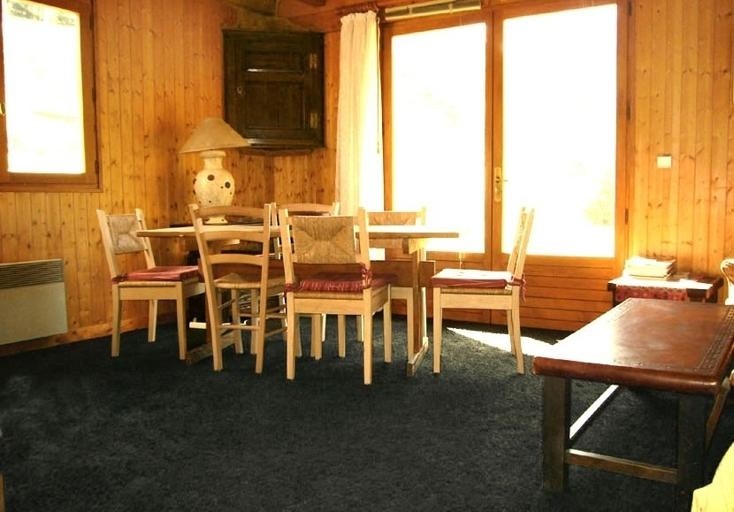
[606,272,726,310]
[221,28,328,156]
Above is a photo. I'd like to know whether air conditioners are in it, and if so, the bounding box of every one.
[0,257,69,346]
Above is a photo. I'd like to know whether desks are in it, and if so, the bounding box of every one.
[532,296,733,512]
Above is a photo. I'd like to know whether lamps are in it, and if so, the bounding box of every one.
[177,113,253,225]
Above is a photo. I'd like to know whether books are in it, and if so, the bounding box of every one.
[621,254,679,283]
